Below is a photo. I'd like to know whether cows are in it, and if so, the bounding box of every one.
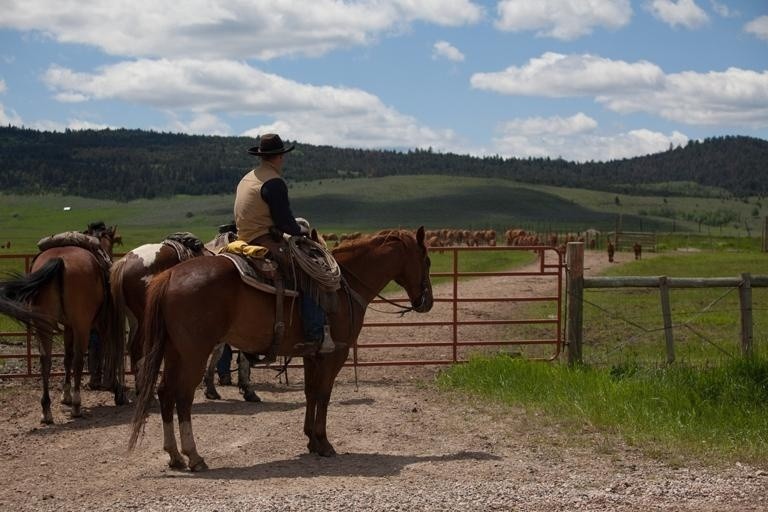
[322,228,498,254]
[634,241,642,260]
[558,244,567,262]
[504,229,596,250]
[608,243,615,262]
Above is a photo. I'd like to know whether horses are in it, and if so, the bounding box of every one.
[108,231,261,408]
[0,220,118,426]
[125,224,434,473]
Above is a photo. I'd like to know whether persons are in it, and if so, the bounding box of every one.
[234,133,348,352]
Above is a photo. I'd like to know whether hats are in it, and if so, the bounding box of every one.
[83,222,107,234]
[247,134,296,157]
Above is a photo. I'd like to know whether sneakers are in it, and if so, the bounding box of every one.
[304,330,347,352]
[86,380,128,390]
[217,375,231,385]
[238,354,261,363]
